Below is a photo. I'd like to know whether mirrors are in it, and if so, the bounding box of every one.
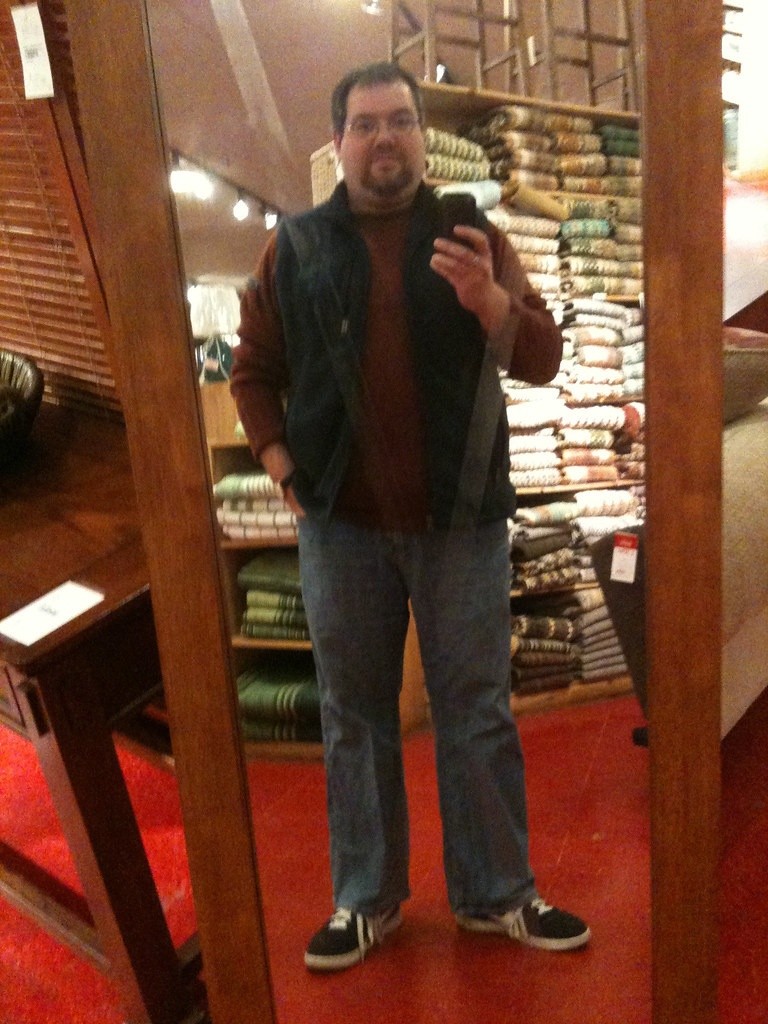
[64,0,721,1024]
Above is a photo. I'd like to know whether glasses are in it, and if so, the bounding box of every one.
[340,114,423,137]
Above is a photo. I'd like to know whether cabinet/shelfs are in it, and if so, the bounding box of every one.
[197,79,643,766]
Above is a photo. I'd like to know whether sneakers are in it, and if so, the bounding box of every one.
[455,892,591,951]
[305,900,405,970]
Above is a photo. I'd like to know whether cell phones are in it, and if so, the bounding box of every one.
[440,194,476,251]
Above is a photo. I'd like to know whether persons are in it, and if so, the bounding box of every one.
[228,61,592,967]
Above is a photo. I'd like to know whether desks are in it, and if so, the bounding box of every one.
[0,402,202,1024]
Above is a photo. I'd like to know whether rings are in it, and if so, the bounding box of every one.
[472,256,480,266]
